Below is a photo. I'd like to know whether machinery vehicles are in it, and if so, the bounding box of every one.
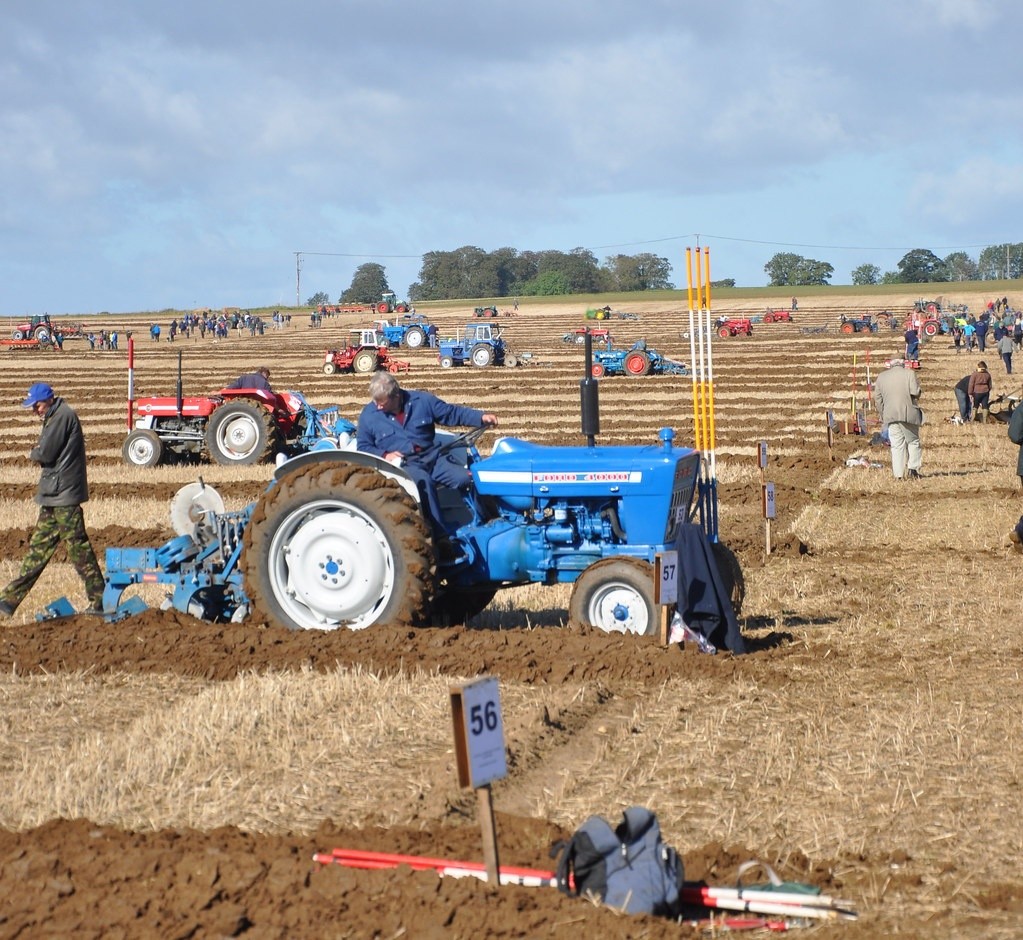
[38,328,745,656]
[591,335,689,379]
[800,297,970,340]
[583,304,640,322]
[1,312,86,350]
[117,333,355,472]
[318,292,412,316]
[472,305,520,319]
[320,328,413,374]
[373,312,440,350]
[681,306,791,338]
[562,322,616,347]
[437,322,540,368]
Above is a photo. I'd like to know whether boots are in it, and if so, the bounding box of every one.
[971,407,978,422]
[984,408,989,424]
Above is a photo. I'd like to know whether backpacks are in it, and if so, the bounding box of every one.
[549,807,685,921]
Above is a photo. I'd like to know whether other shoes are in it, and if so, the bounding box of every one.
[85,604,103,614]
[0,599,15,619]
[1010,531,1023,554]
[908,469,921,478]
[434,536,458,558]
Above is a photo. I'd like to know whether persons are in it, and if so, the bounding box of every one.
[223,367,273,393]
[179,313,199,334]
[719,296,1023,554]
[371,303,375,313]
[605,305,611,319]
[232,311,263,336]
[311,305,339,326]
[427,323,437,348]
[199,311,228,338]
[170,320,178,335]
[513,299,519,310]
[405,305,408,311]
[702,296,706,308]
[628,337,647,352]
[89,332,95,348]
[272,310,291,330]
[0,382,106,616]
[57,333,63,349]
[99,330,118,349]
[356,372,499,522]
[150,322,160,342]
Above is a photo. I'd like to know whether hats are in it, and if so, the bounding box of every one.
[21,383,53,407]
